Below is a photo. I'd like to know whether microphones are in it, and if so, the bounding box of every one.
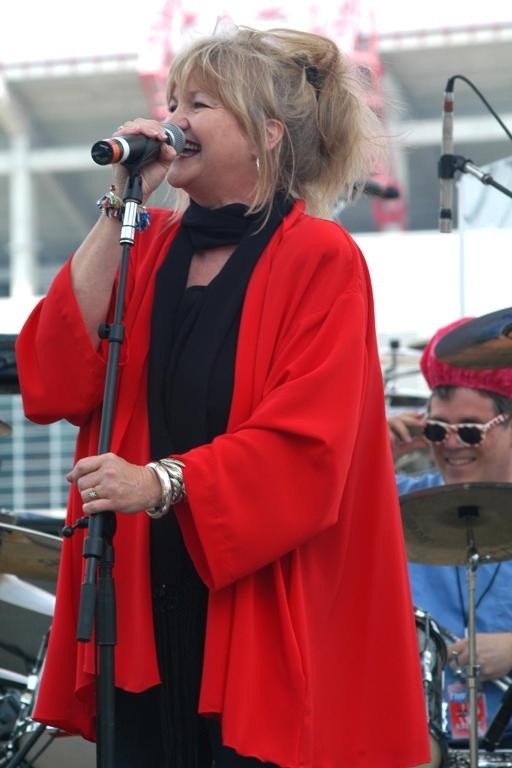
[438,78,455,234]
[91,123,185,166]
[353,179,400,199]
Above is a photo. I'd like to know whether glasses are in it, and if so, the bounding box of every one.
[418,411,511,449]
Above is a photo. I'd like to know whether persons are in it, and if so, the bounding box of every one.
[14,25,432,766]
[385,317,512,742]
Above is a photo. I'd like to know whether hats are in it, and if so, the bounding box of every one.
[421,308,512,399]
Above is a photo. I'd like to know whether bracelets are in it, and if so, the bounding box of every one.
[96,184,150,232]
[144,461,172,520]
[159,457,186,504]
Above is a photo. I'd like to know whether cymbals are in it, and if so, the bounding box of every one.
[398,485,512,563]
[0,522,64,580]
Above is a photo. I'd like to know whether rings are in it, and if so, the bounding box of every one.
[117,125,124,130]
[87,486,97,499]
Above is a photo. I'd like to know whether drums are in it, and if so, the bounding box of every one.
[17,699,99,764]
[408,605,448,768]
